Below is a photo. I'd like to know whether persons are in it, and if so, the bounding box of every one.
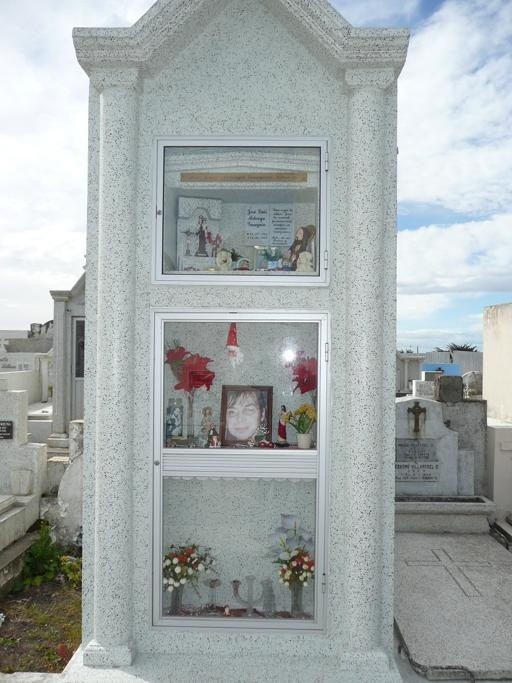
[224,388,268,444]
[277,404,289,442]
[284,225,312,270]
[200,405,215,439]
[167,407,183,436]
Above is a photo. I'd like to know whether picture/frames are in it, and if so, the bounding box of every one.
[221,386,273,447]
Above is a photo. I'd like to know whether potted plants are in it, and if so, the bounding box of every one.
[231,249,242,268]
[263,248,282,268]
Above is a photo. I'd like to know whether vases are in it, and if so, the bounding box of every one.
[289,584,304,618]
[297,434,311,449]
[171,585,183,614]
[186,388,194,438]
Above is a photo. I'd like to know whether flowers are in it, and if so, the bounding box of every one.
[280,404,318,434]
[272,522,314,587]
[162,539,217,598]
[163,340,214,417]
[286,351,317,403]
